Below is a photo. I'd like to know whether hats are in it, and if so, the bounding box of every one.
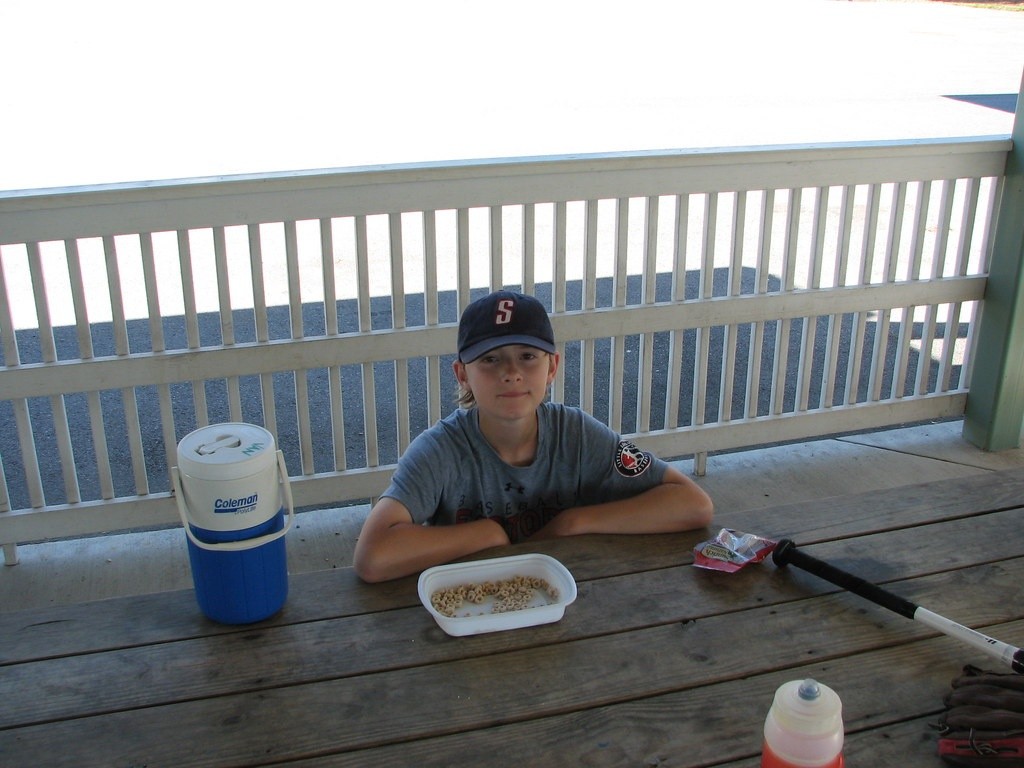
[458,289,556,365]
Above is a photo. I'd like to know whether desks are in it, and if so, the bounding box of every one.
[0,468,1024,768]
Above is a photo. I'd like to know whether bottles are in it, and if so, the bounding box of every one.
[761,679,844,768]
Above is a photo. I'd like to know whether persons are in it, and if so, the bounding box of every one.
[354,289,714,582]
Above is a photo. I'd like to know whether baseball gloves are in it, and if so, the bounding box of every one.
[928,664,1024,768]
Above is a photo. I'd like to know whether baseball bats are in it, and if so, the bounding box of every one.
[771,537,1024,676]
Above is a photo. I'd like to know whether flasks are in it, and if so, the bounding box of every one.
[172,423,294,625]
